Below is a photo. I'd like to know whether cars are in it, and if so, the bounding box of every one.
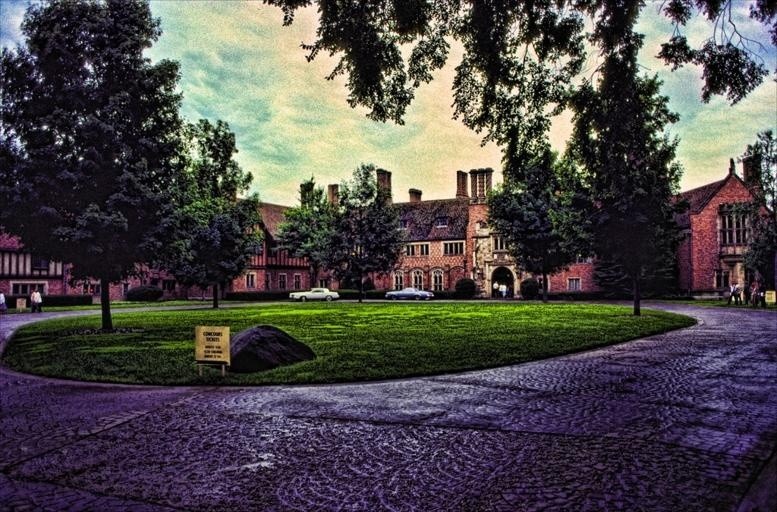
[385,286,433,300]
[289,287,340,302]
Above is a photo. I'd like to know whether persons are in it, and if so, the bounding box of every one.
[34,289,42,312]
[30,289,36,313]
[0,289,8,315]
[491,279,514,299]
[726,279,767,310]
